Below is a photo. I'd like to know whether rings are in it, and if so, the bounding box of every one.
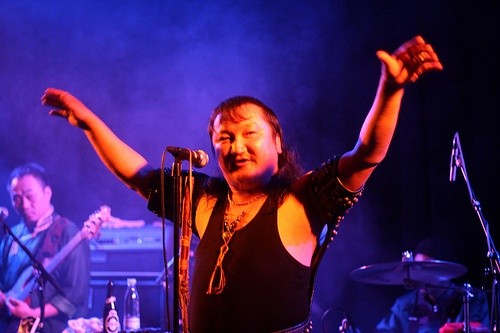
[416,53,425,61]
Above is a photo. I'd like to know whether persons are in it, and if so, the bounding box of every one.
[42,36,442,333]
[0,160,88,333]
[416,232,441,261]
[100,177,166,328]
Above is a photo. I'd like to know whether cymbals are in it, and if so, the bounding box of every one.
[347,252,468,286]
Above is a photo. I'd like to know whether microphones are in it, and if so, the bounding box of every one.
[450,136,459,181]
[341,312,361,333]
[167,147,209,168]
[0,207,9,222]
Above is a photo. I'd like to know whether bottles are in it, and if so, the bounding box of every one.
[123,278,141,332]
[103,281,122,333]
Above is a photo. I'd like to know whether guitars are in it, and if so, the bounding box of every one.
[0,203,111,333]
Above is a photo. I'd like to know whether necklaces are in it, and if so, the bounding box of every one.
[207,191,265,294]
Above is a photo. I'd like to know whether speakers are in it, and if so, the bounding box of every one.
[85,279,175,333]
